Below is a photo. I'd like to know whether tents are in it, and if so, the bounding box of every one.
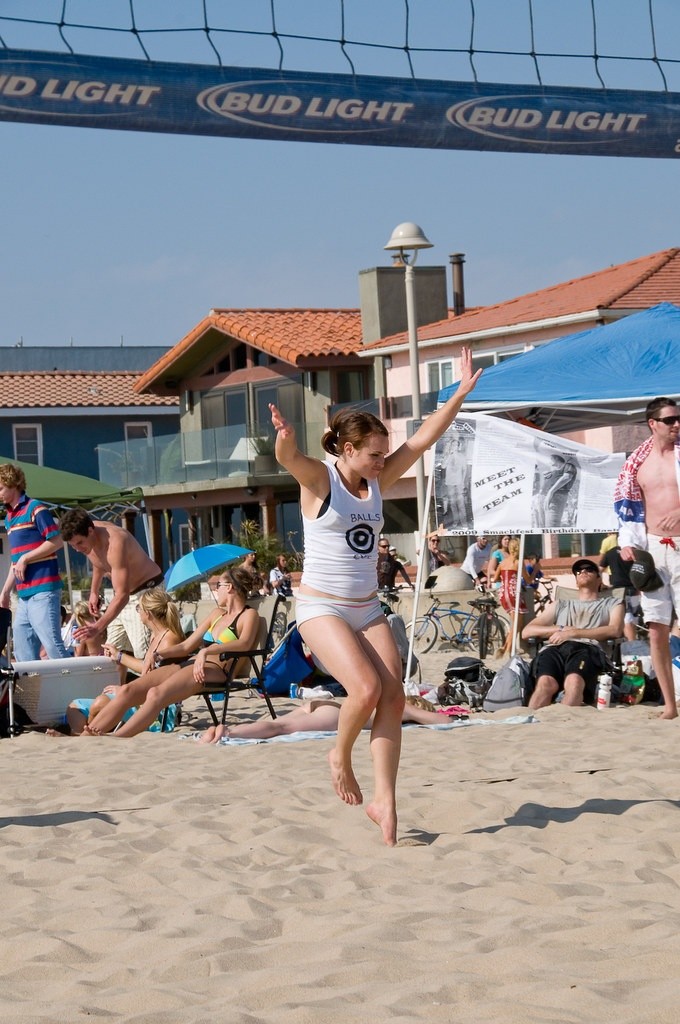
[254,619,318,695]
[0,455,155,561]
[412,302,680,592]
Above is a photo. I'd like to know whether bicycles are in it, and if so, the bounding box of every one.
[474,588,506,659]
[378,585,404,615]
[405,583,512,654]
[533,579,554,615]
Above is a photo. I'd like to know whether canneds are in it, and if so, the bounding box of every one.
[290,683,298,698]
[70,626,81,646]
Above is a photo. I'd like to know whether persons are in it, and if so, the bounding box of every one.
[520,531,644,710]
[533,454,576,528]
[238,551,266,597]
[440,438,468,530]
[416,534,449,589]
[0,462,70,661]
[268,554,294,596]
[67,588,187,735]
[45,567,260,739]
[266,345,483,851]
[60,595,107,657]
[298,601,418,698]
[375,538,414,597]
[621,392,680,719]
[459,535,544,656]
[58,508,167,673]
[204,695,453,744]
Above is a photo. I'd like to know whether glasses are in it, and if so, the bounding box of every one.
[432,539,440,542]
[216,582,230,590]
[655,415,680,425]
[482,538,486,540]
[379,545,390,548]
[576,566,594,575]
[391,554,397,558]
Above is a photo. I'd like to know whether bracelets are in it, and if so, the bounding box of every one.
[116,651,122,665]
[559,625,564,632]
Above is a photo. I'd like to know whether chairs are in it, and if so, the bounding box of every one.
[527,585,624,704]
[160,593,285,734]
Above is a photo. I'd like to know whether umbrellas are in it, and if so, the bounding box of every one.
[165,543,256,606]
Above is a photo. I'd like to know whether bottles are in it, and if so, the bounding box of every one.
[596,675,612,711]
[71,626,81,647]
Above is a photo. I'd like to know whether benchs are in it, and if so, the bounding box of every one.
[184,436,270,477]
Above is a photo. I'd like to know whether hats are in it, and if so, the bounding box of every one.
[571,559,599,575]
[630,551,662,591]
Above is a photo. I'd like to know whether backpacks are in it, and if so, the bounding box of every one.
[483,654,530,714]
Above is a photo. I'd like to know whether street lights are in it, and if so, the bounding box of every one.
[384,222,434,582]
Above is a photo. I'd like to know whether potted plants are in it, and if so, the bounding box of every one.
[247,431,276,475]
[72,577,103,606]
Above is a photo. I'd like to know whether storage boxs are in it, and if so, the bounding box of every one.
[11,655,124,727]
